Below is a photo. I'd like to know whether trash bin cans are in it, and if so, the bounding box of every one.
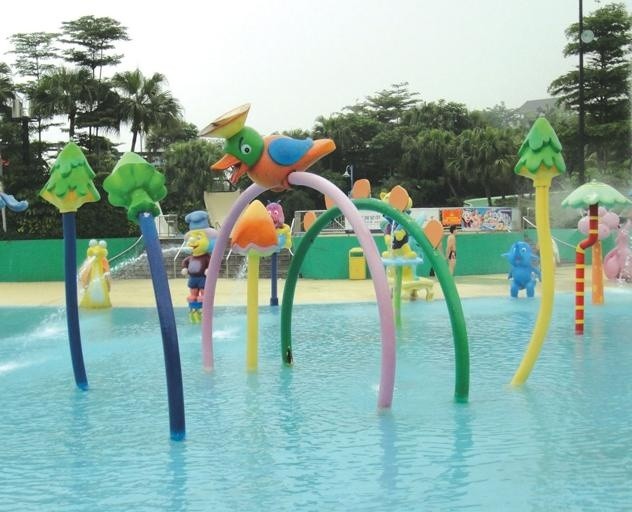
[349,247,367,280]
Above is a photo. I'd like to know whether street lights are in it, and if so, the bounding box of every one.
[575,0,597,185]
[8,98,42,181]
[342,164,355,189]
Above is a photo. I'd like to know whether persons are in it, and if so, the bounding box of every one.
[445,225,457,275]
